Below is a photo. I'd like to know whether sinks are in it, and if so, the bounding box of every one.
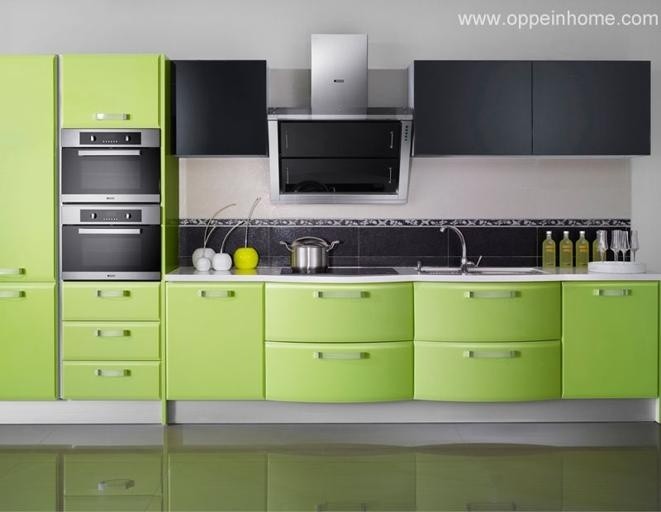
[465,267,546,280]
[413,268,466,279]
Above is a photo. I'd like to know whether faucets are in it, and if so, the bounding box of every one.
[440,226,467,268]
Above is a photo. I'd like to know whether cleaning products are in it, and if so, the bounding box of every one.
[542,230,557,268]
[559,231,573,268]
[576,231,589,268]
[593,239,606,261]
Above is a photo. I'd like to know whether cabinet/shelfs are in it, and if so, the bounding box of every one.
[51,54,178,275]
[56,282,165,425]
[558,282,661,423]
[1,446,661,510]
[1,56,56,430]
[259,283,415,426]
[159,280,264,424]
[408,282,561,424]
[161,59,270,156]
[409,60,653,159]
